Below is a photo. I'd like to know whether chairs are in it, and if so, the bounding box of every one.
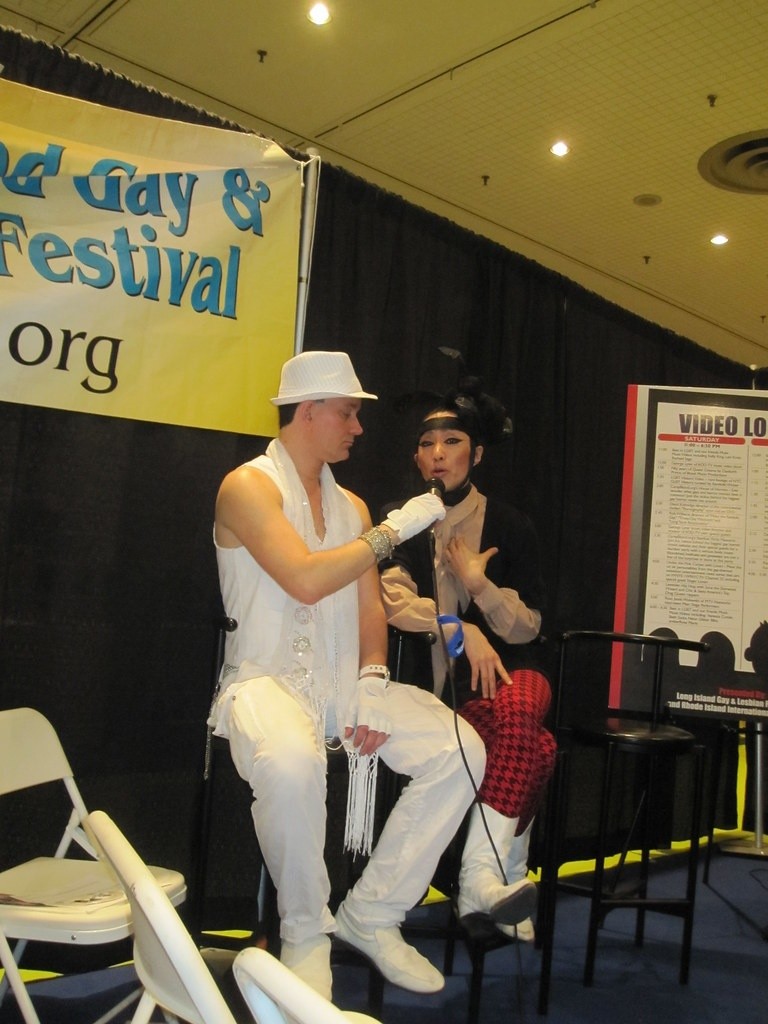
[0,706,381,1024]
[191,614,708,1024]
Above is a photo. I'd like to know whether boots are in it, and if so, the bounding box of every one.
[459,803,537,947]
[497,817,535,943]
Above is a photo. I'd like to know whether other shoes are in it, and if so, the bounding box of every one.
[278,935,333,1024]
[330,902,444,992]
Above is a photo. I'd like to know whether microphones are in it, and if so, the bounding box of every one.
[425,477,446,535]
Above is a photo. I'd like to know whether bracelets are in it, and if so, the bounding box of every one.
[358,525,395,563]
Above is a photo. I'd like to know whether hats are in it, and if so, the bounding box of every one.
[270,352,378,406]
[379,391,514,477]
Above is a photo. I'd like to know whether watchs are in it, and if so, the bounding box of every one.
[359,665,391,688]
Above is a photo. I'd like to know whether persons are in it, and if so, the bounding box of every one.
[206,350,487,1002]
[376,346,558,941]
